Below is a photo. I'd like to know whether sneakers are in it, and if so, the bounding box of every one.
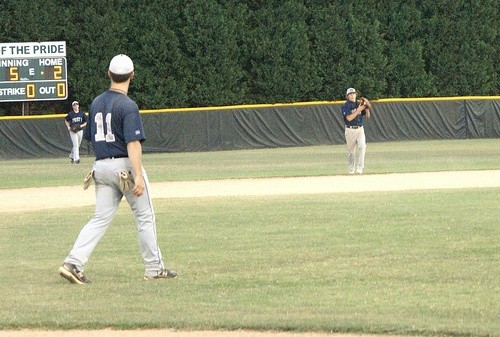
[143,270,177,281]
[58,262,92,285]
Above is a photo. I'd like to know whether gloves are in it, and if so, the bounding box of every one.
[83,170,94,191]
[119,170,135,193]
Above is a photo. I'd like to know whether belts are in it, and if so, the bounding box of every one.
[346,125,361,129]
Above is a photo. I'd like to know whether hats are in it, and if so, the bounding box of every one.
[109,54,134,74]
[346,87,355,97]
[71,100,79,106]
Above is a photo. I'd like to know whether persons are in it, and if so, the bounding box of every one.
[341,88,371,174]
[57,54,178,286]
[64,100,88,164]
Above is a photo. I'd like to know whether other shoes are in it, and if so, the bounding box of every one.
[349,166,362,175]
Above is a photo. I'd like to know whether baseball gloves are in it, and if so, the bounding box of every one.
[359,96,372,108]
